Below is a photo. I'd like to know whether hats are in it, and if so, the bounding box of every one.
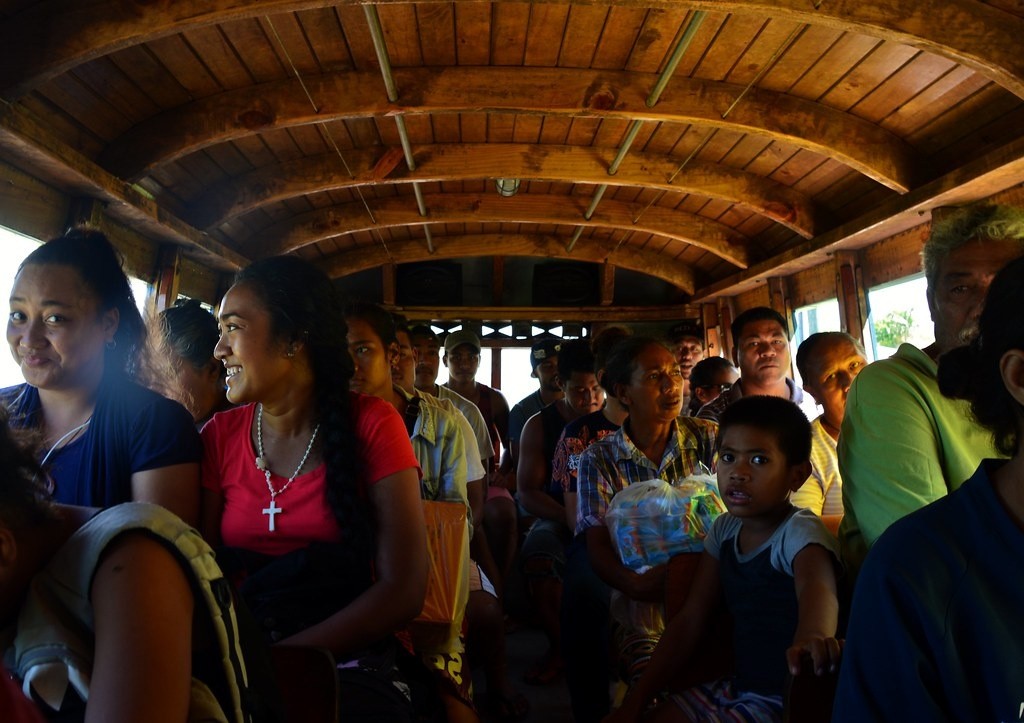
[444,330,481,353]
[530,337,564,369]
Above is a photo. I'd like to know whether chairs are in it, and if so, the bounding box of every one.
[417,498,469,626]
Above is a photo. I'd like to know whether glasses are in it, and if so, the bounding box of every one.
[700,383,733,394]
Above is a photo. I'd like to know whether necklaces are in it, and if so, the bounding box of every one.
[36,414,93,499]
[256,404,320,530]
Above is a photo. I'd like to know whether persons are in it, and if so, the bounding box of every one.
[200,254,480,723]
[1,405,255,723]
[832,203,1024,723]
[135,291,861,718]
[1,221,204,534]
[606,395,847,723]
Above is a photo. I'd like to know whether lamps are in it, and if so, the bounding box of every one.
[495,179,521,196]
